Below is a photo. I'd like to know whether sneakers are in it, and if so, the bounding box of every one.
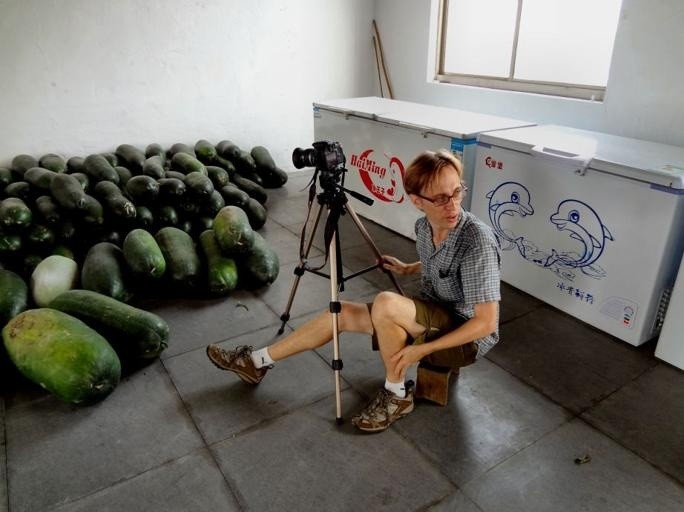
[349,379,416,434]
[206,343,276,387]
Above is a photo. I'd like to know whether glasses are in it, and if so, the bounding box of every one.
[417,182,469,207]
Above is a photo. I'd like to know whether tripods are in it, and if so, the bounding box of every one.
[273,191,407,426]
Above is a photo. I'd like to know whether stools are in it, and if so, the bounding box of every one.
[414,362,458,405]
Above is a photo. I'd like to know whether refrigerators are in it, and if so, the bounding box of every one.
[468,124,684,348]
[655,252,683,371]
[312,96,537,244]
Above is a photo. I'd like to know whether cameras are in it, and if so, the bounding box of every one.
[291,139,346,173]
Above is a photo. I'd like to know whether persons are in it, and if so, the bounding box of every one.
[206,151,502,433]
